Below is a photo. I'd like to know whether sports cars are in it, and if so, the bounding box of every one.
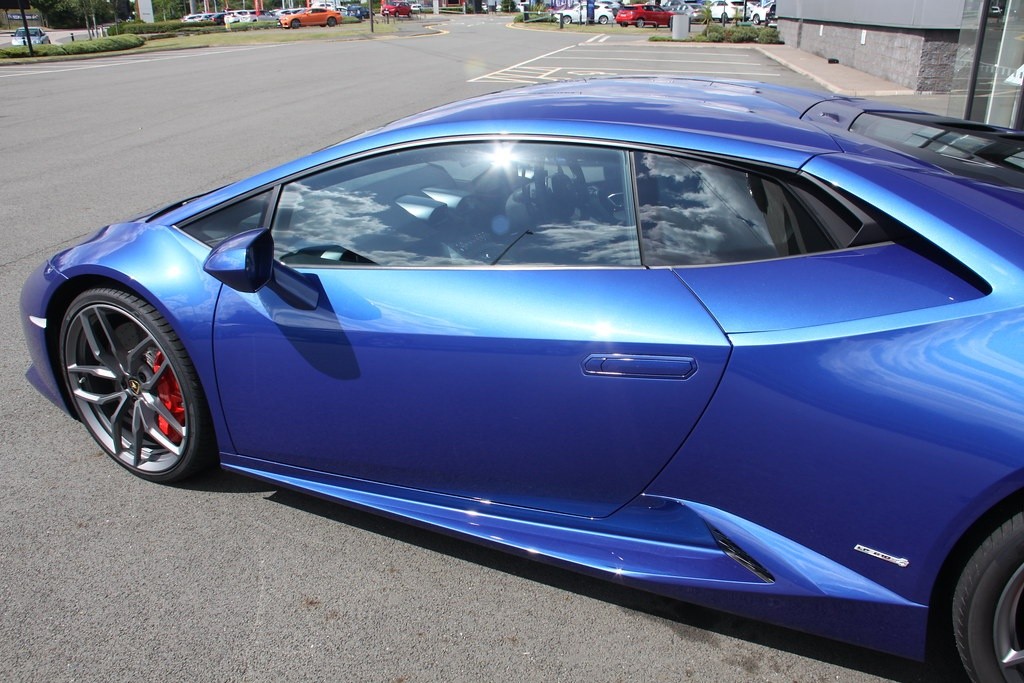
[19,75,1024,683]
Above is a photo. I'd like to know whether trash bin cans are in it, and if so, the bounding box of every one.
[672,14,690,40]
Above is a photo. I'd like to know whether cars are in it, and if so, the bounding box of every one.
[663,0,776,27]
[270,2,376,29]
[551,0,621,25]
[381,1,423,17]
[11,27,51,46]
[181,9,282,28]
[616,4,677,28]
